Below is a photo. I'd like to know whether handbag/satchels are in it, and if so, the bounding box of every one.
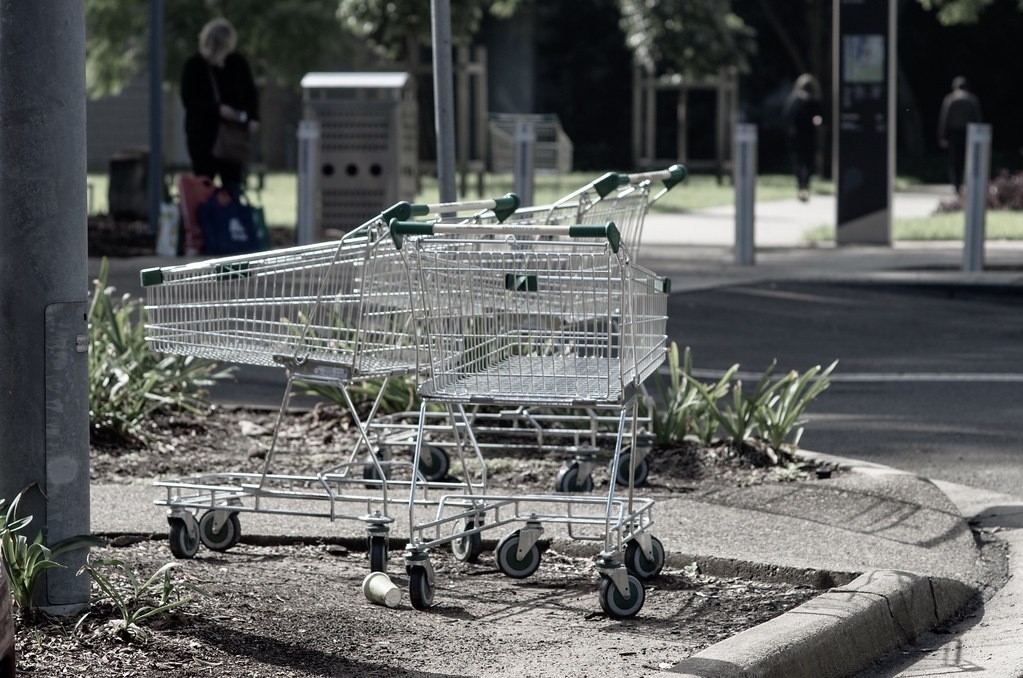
[177,174,271,260]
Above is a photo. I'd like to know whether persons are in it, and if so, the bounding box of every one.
[938,77,981,194]
[784,74,824,200]
[179,17,261,194]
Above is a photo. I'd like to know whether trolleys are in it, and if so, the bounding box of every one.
[141,164,688,621]
[206,182,274,249]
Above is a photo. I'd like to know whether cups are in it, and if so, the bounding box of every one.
[362,571,401,607]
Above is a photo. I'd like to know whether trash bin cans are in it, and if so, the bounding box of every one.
[301,71,419,243]
